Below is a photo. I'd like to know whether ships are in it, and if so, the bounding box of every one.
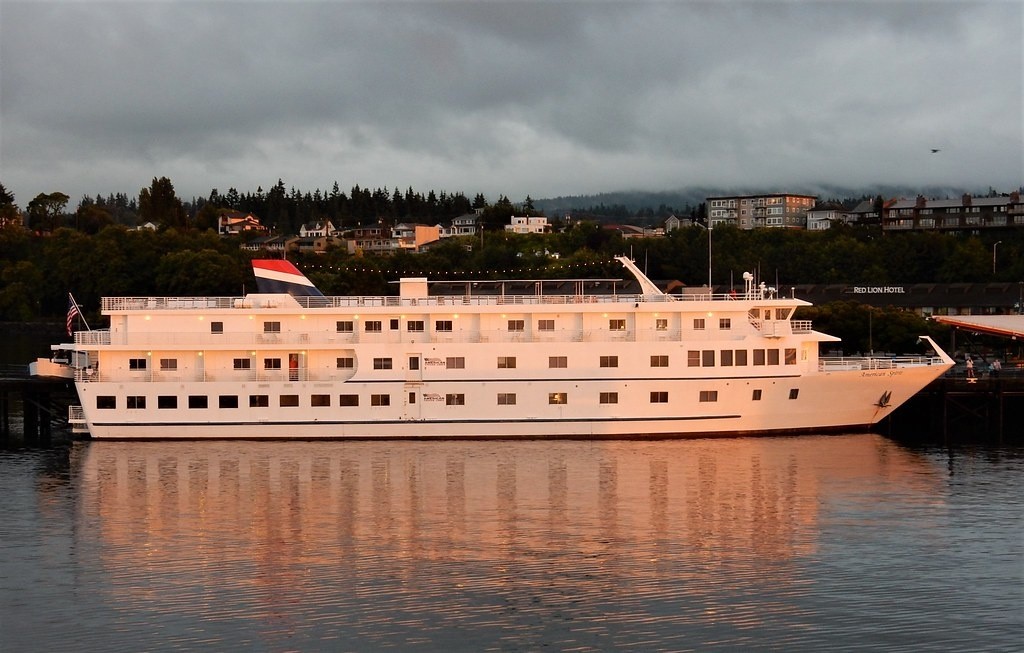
[29,199,957,437]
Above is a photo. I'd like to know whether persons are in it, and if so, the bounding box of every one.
[992,359,1002,372]
[289,356,299,382]
[966,356,976,378]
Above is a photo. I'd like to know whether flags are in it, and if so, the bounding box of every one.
[66,296,80,336]
